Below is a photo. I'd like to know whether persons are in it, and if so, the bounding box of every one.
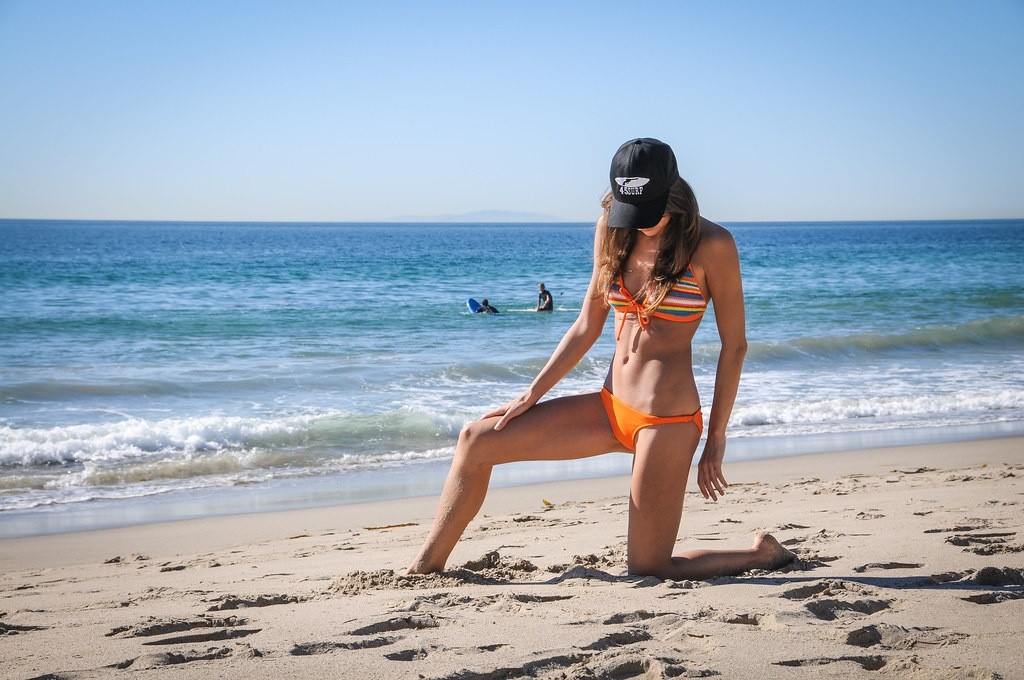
[476,299,500,313]
[535,283,553,311]
[393,138,800,581]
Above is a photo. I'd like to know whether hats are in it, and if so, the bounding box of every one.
[607,137,679,228]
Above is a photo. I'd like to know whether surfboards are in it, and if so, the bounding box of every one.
[467,297,482,313]
